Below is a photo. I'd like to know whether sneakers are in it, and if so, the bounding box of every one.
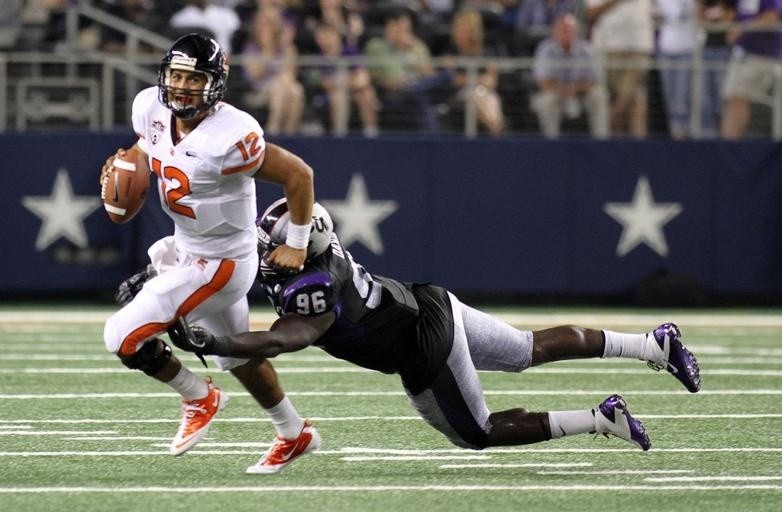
[647,322,700,392]
[169,376,230,457]
[244,418,322,476]
[589,394,651,452]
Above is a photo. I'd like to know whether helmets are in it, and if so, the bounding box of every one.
[157,32,230,120]
[256,194,334,285]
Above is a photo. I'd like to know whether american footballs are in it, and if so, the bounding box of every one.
[102,149,150,224]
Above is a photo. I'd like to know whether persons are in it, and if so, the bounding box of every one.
[1,0,781,141]
[99,32,324,476]
[113,195,702,455]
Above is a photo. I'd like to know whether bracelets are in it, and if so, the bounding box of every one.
[285,218,313,251]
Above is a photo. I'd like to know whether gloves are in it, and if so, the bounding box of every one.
[168,314,217,369]
[112,264,157,307]
